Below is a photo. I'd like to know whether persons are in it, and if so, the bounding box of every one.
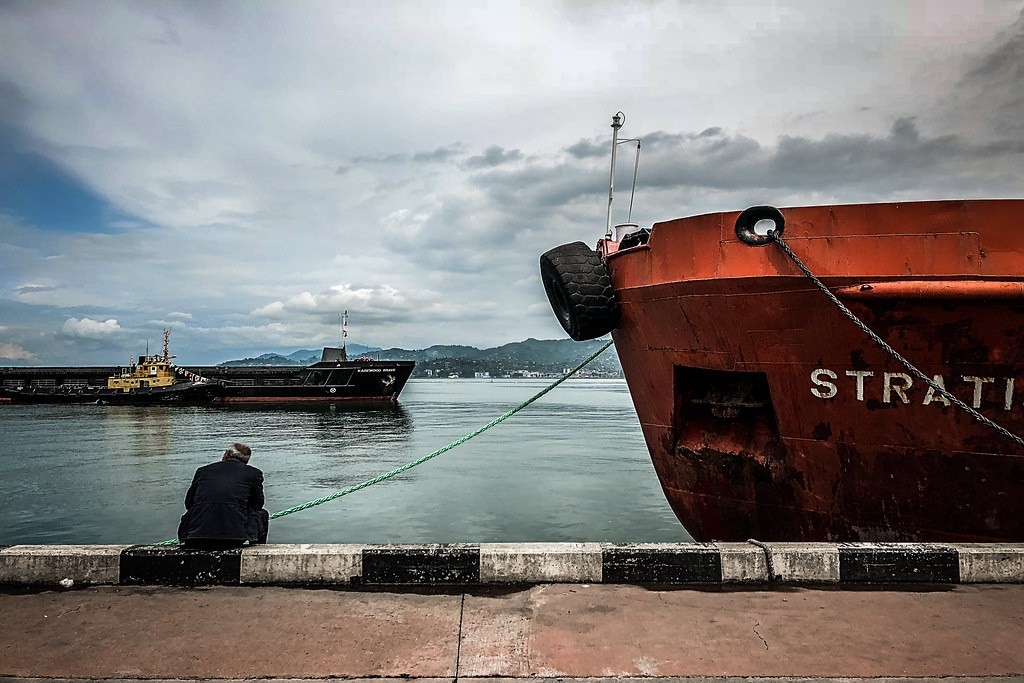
[177,443,269,549]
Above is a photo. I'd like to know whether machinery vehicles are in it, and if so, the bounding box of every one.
[107,324,177,393]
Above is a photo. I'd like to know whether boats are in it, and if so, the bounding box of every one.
[0,309,418,408]
[537,108,1024,546]
